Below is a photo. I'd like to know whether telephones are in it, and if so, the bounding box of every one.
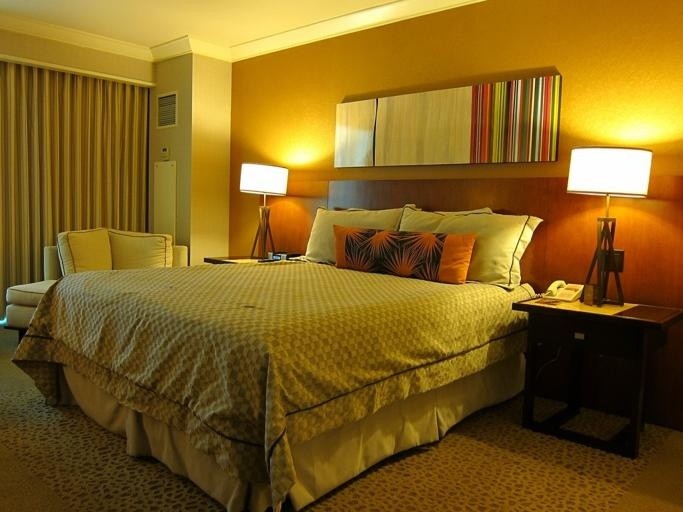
[541,277,583,303]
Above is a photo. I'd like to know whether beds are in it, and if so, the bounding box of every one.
[12,203,544,511]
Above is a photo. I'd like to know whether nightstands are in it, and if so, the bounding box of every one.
[203,255,269,265]
[510,293,683,462]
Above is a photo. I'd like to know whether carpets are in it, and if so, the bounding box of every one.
[0,383,674,511]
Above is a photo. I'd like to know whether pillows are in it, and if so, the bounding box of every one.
[301,203,422,266]
[107,226,173,270]
[396,203,545,292]
[332,224,479,285]
[54,228,116,278]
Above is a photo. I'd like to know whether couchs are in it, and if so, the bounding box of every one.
[3,227,189,345]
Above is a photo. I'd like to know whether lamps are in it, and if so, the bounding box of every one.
[565,144,654,309]
[239,162,289,261]
